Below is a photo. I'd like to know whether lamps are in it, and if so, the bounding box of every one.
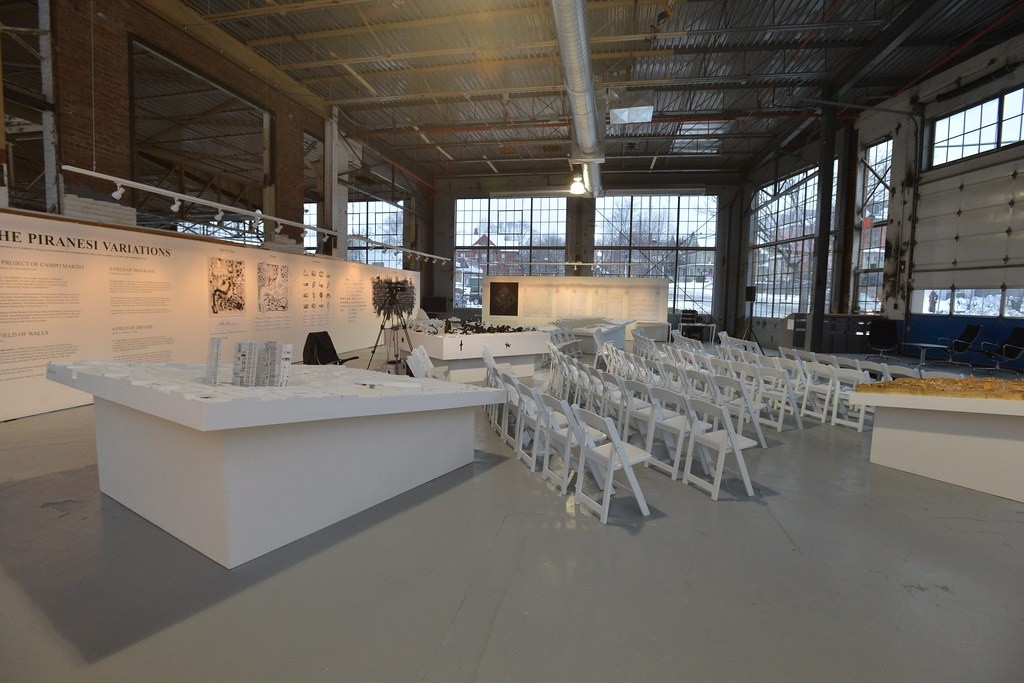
[570,169,587,195]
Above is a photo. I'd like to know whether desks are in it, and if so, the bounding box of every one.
[677,321,716,345]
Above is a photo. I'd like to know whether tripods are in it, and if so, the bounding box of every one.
[742,302,765,356]
[366,295,413,371]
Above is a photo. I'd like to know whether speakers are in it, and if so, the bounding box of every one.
[745,286,756,301]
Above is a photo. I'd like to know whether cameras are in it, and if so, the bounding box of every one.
[384,282,407,300]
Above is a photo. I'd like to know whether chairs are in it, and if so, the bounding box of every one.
[865,319,900,364]
[303,331,360,368]
[979,326,1024,378]
[405,344,449,381]
[482,327,966,526]
[930,324,983,368]
[680,308,705,344]
